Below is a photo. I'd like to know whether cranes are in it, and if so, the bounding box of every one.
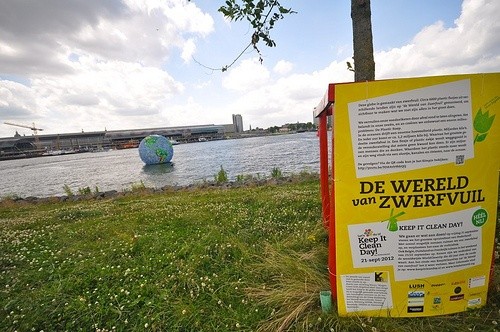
[3,122,43,136]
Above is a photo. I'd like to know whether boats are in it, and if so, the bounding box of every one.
[0,131,138,160]
[168,131,226,145]
